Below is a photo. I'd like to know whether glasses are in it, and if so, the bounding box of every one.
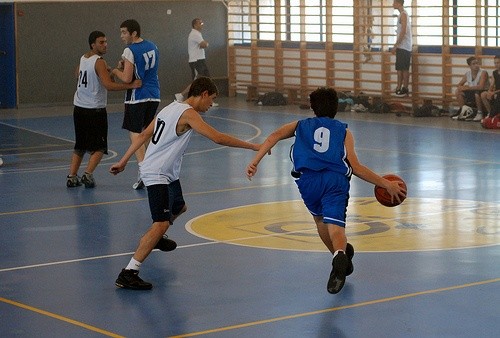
[198,22,204,26]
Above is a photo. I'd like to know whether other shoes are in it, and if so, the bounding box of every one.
[173,93,184,102]
[451,114,459,120]
[132,167,145,190]
[212,102,219,106]
[473,111,483,121]
[327,251,348,294]
[344,243,354,276]
[393,87,409,97]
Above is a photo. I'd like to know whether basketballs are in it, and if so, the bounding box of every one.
[374,175,407,207]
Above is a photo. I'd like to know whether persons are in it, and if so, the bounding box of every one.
[451,57,490,121]
[481,54,500,123]
[108,76,271,291]
[66,31,142,188]
[246,87,406,295]
[391,0,412,98]
[175,18,220,107]
[110,19,161,190]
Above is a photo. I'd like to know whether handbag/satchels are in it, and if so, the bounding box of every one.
[482,114,500,129]
[457,104,473,121]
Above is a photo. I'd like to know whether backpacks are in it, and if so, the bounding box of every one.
[335,92,448,118]
[256,91,288,106]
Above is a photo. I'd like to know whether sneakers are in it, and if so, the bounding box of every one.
[153,236,177,252]
[81,173,96,188]
[66,175,82,187]
[115,268,152,290]
